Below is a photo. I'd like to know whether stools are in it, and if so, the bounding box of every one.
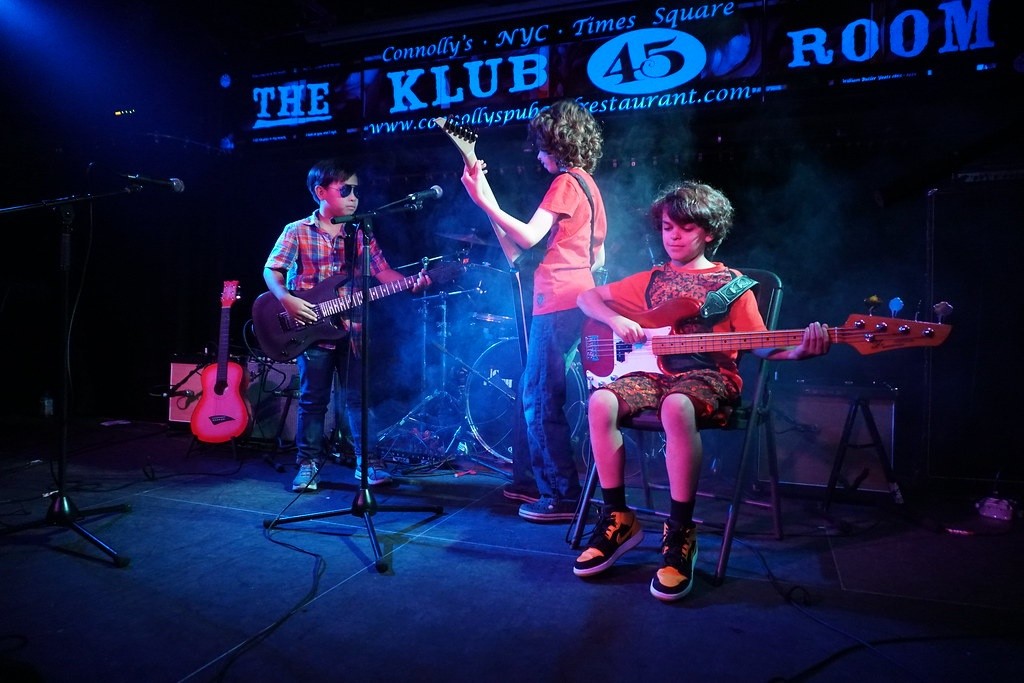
[262,374,340,473]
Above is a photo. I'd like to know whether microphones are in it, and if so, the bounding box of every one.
[125,174,185,194]
[408,186,443,201]
[162,390,194,398]
[272,391,300,399]
[792,423,821,432]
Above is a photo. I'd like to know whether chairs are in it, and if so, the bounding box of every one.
[570,268,784,586]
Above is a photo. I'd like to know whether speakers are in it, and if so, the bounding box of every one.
[237,356,337,450]
[167,354,208,429]
[922,185,1024,490]
[755,375,905,497]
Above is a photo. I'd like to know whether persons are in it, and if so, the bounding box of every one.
[263,155,432,493]
[573,181,830,601]
[461,100,608,523]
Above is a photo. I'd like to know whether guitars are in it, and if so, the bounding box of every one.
[253,261,460,363]
[191,281,252,443]
[579,297,952,387]
[434,118,543,367]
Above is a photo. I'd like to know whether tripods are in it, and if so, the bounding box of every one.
[0,182,145,569]
[264,245,487,571]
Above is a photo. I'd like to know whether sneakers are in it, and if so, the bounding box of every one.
[573,505,643,576]
[650,516,698,599]
[503,483,540,503]
[292,462,317,490]
[354,461,393,485]
[518,497,583,519]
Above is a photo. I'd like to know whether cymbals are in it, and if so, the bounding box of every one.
[434,230,498,249]
[470,263,507,279]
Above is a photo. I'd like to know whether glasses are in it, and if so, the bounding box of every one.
[324,184,363,200]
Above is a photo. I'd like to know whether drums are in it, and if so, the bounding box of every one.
[466,338,586,463]
[593,268,606,284]
[463,313,515,369]
